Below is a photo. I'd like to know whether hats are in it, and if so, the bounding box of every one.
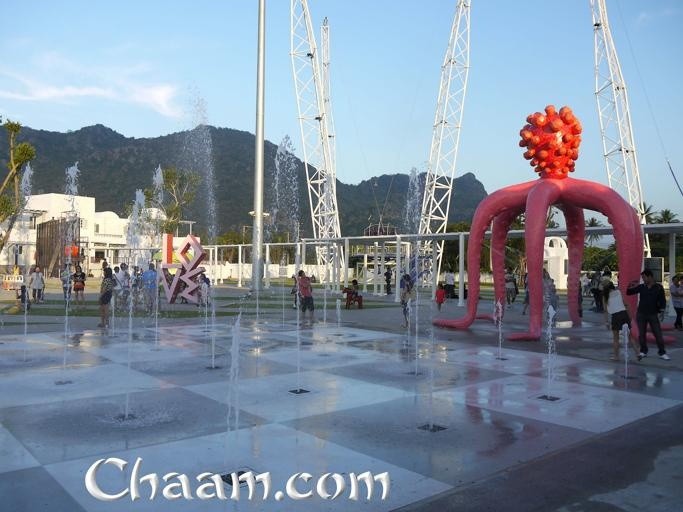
[602,279,609,287]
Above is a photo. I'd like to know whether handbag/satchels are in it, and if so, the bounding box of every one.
[590,288,599,294]
[74,283,84,289]
[101,291,112,306]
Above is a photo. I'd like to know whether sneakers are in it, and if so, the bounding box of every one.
[640,352,647,358]
[659,354,670,360]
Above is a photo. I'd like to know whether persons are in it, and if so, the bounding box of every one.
[348,279,358,304]
[504,267,516,309]
[342,287,362,310]
[445,269,455,299]
[398,273,412,329]
[98,259,208,315]
[382,267,393,295]
[71,265,87,309]
[625,269,672,361]
[541,266,620,327]
[27,266,45,304]
[669,275,682,332]
[520,273,529,316]
[433,281,445,311]
[599,278,644,361]
[13,284,31,313]
[295,269,318,324]
[59,263,74,303]
[95,267,112,327]
